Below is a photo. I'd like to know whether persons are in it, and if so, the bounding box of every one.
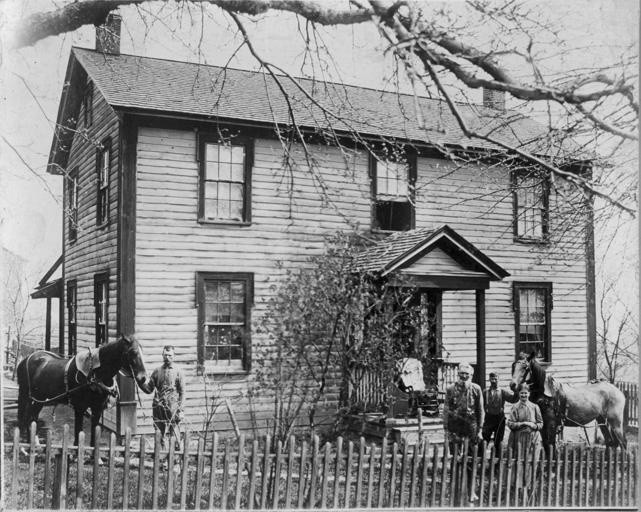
[140,345,185,470]
[443,363,486,501]
[477,372,520,475]
[507,383,544,499]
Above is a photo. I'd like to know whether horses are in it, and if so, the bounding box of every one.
[510,348,626,481]
[16,333,155,466]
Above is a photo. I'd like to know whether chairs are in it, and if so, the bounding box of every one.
[384,380,409,421]
[396,357,439,417]
